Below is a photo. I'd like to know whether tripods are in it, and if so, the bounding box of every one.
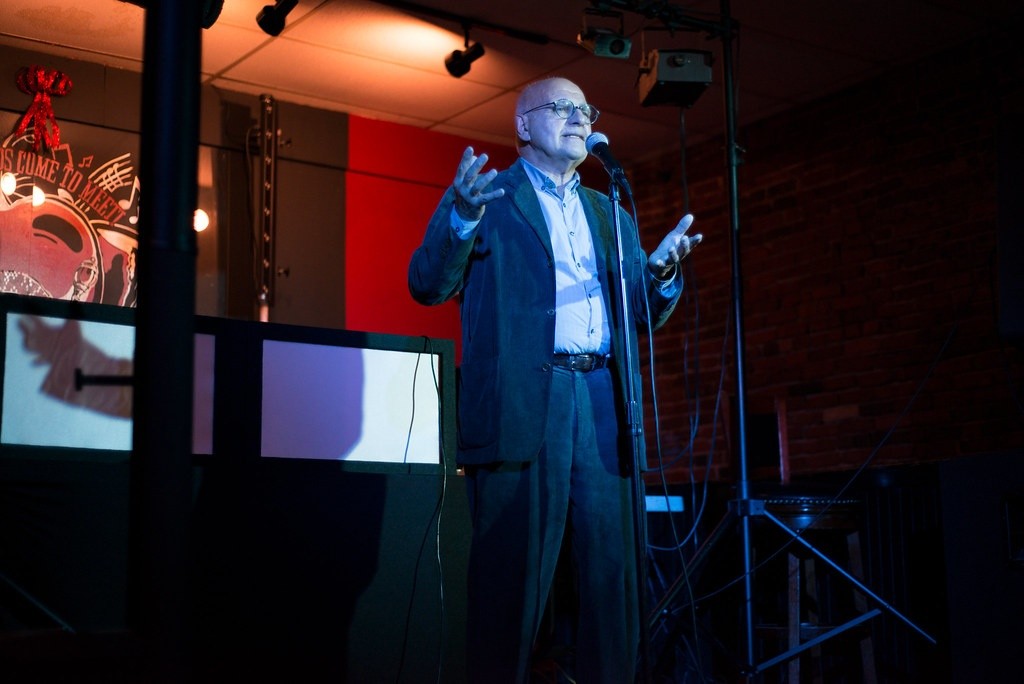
[639,37,939,684]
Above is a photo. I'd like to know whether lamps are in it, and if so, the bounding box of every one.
[575,7,632,59]
[256,0,300,37]
[444,40,486,79]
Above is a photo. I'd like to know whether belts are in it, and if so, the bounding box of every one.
[554,352,608,372]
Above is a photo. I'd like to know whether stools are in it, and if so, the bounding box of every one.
[736,492,880,684]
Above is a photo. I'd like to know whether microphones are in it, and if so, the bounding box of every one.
[585,132,631,195]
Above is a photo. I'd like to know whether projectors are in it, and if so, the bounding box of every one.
[577,28,630,58]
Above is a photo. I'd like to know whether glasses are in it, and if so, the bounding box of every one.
[522,99,601,125]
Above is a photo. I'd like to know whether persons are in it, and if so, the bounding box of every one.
[408,77,702,684]
